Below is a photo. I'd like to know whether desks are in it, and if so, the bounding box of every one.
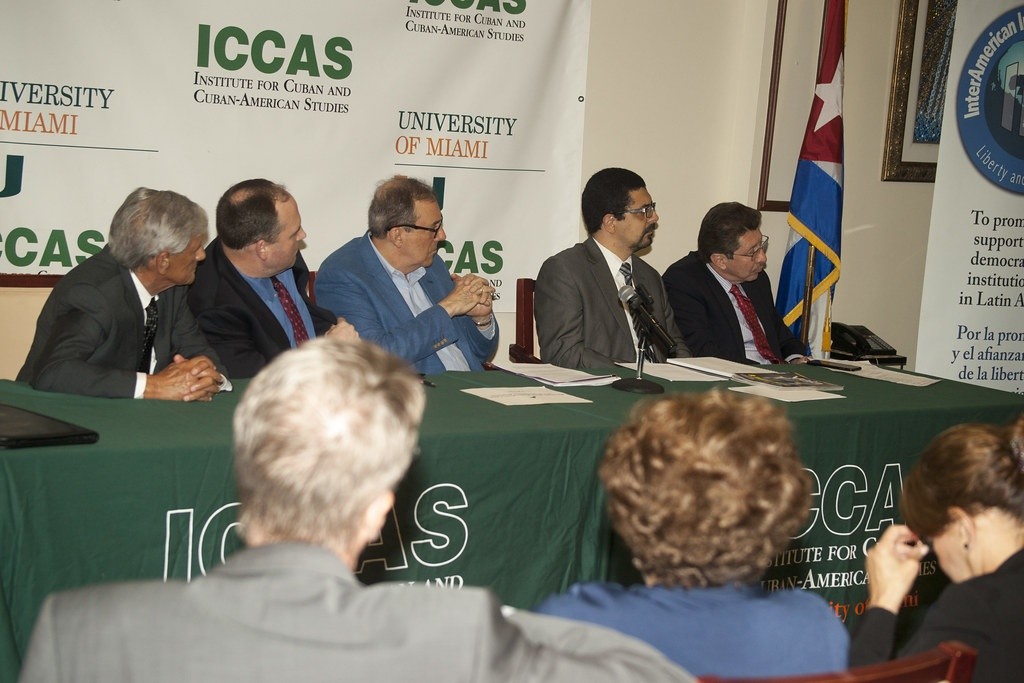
[0,363,1024,683]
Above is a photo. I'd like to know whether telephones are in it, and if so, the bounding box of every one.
[830,321,897,356]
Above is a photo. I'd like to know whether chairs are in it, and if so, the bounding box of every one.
[509,278,544,364]
[699,637,981,683]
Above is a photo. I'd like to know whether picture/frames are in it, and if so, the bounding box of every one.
[876,0,957,185]
[757,1,828,212]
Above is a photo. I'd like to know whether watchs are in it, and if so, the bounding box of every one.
[471,316,493,328]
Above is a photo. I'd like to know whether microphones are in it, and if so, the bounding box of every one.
[618,284,677,356]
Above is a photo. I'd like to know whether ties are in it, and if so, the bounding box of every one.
[730,284,781,364]
[619,262,660,363]
[270,275,310,350]
[137,297,159,375]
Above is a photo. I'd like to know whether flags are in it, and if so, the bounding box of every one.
[771,0,848,361]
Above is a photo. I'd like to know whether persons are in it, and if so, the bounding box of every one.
[12,185,235,404]
[528,387,851,676]
[15,332,700,682]
[662,200,815,366]
[312,176,500,375]
[850,412,1023,682]
[185,177,364,382]
[533,166,694,368]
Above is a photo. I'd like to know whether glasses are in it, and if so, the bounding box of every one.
[724,235,769,261]
[386,215,444,239]
[608,202,656,218]
